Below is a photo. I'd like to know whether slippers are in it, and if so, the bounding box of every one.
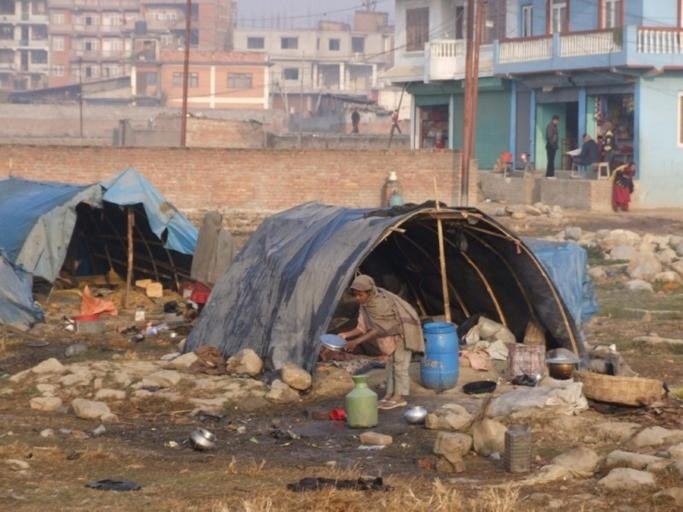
[378,399,408,410]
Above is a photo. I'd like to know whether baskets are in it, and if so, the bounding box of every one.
[574,370,664,407]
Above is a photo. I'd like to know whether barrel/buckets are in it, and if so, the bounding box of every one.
[389,188,403,207]
[420,322,460,389]
[505,424,532,473]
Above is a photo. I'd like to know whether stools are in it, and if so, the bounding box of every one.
[572,162,590,178]
[592,162,610,181]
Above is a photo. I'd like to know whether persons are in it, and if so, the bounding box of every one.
[391,109,401,135]
[351,108,360,132]
[612,162,635,211]
[572,134,600,165]
[546,116,560,179]
[336,275,425,411]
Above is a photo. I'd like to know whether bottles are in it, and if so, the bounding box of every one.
[134,304,144,322]
[380,168,404,210]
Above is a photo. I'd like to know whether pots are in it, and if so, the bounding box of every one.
[187,427,219,452]
[403,405,428,424]
[547,358,579,379]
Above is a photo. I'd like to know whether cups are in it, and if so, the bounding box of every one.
[329,407,346,419]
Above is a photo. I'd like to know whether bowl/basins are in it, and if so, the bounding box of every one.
[463,380,497,394]
[71,313,100,323]
[317,332,353,354]
